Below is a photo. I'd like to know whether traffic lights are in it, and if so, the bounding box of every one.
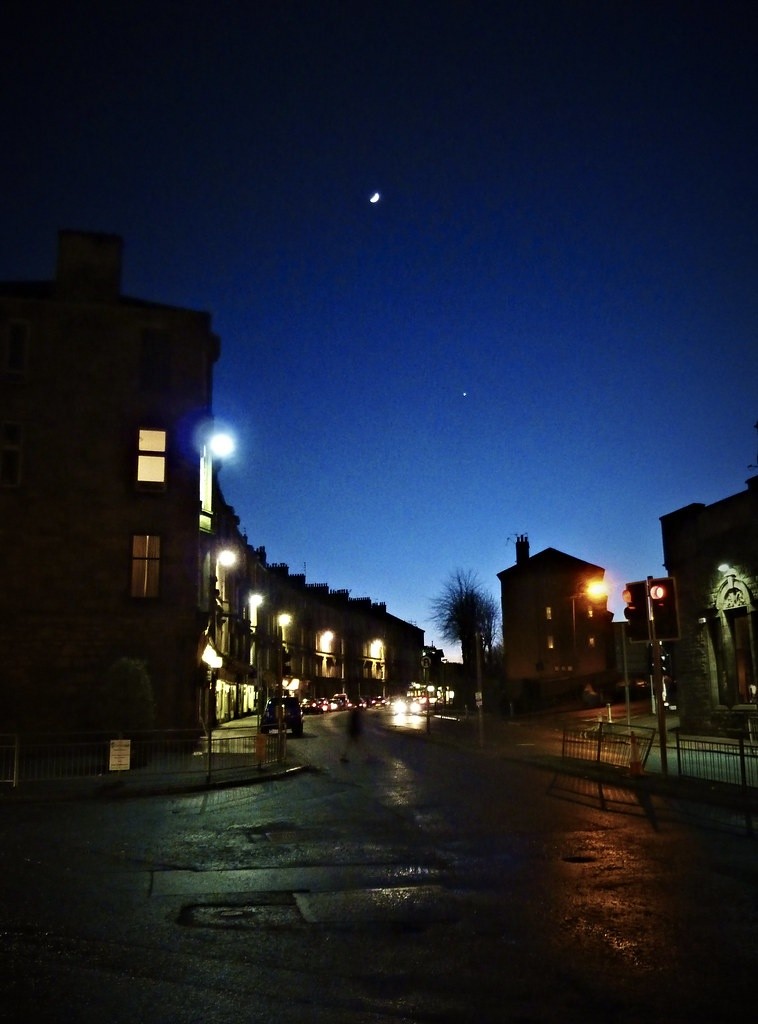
[620,576,650,642]
[647,579,678,641]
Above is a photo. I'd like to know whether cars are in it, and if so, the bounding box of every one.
[300,693,443,716]
[259,695,304,736]
